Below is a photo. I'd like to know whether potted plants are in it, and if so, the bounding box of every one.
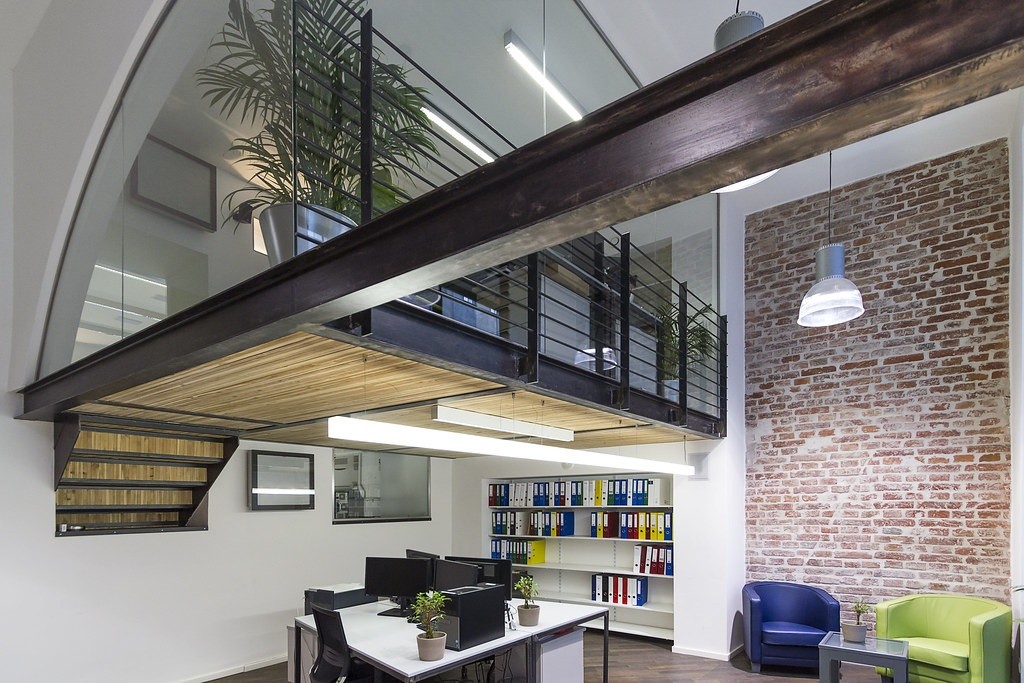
[404,591,447,661]
[841,600,875,642]
[655,304,718,404]
[193,0,440,269]
[511,576,540,626]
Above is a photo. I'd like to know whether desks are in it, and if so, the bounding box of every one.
[294,600,610,683]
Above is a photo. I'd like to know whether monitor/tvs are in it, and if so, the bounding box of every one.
[365,548,512,618]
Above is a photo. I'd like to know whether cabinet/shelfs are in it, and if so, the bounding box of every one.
[485,481,676,643]
[286,626,319,683]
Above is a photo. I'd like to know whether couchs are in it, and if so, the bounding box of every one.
[876,594,1013,683]
[742,582,842,674]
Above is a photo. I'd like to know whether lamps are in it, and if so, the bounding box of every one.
[797,151,864,326]
[575,309,617,372]
[418,106,496,167]
[327,354,699,480]
[714,0,783,53]
[503,30,587,122]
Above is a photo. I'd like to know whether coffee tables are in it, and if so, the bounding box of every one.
[819,631,909,683]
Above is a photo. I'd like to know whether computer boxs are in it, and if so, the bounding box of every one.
[432,582,506,652]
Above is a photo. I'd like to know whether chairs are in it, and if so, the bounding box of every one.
[306,601,376,683]
[511,574,533,598]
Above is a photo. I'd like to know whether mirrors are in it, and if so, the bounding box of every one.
[332,447,432,524]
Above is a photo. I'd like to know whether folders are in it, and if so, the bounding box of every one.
[633,544,672,575]
[592,573,648,606]
[491,509,575,537]
[491,538,546,566]
[487,478,650,506]
[591,511,673,540]
